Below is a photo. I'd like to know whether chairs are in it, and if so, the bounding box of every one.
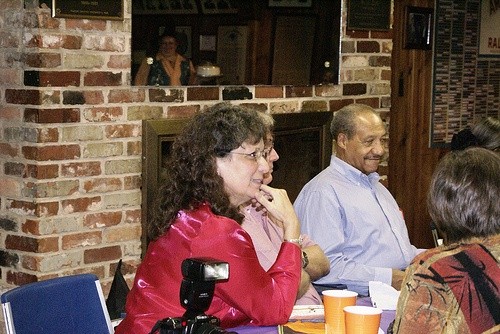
[1,274,115,334]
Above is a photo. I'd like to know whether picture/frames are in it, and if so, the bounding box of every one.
[402,5,434,50]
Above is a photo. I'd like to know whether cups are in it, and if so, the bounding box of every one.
[322,290,358,334]
[344,306,383,334]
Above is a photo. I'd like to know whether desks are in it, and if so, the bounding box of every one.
[224,295,397,334]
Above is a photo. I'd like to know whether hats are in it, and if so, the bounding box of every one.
[450,118,500,150]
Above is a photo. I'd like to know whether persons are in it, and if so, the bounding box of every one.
[294,104,429,296]
[134,31,203,86]
[115,101,330,334]
[392,118,500,334]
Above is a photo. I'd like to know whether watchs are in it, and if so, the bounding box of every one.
[300,250,309,269]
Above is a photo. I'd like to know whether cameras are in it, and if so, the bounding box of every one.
[158,254,238,334]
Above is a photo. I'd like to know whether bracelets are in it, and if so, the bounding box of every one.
[284,239,302,244]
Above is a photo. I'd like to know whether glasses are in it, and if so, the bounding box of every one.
[228,151,267,161]
[265,143,275,154]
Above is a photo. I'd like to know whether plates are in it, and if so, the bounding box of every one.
[193,73,223,78]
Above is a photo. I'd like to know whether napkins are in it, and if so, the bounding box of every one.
[368,281,400,310]
